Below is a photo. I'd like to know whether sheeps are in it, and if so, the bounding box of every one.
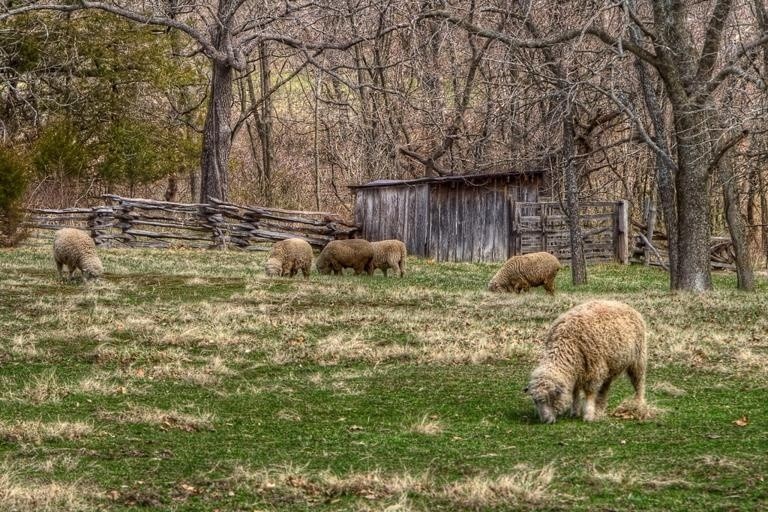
[365,239,407,279]
[262,238,314,280]
[316,239,379,276]
[528,300,646,424]
[53,228,105,285]
[488,252,563,296]
